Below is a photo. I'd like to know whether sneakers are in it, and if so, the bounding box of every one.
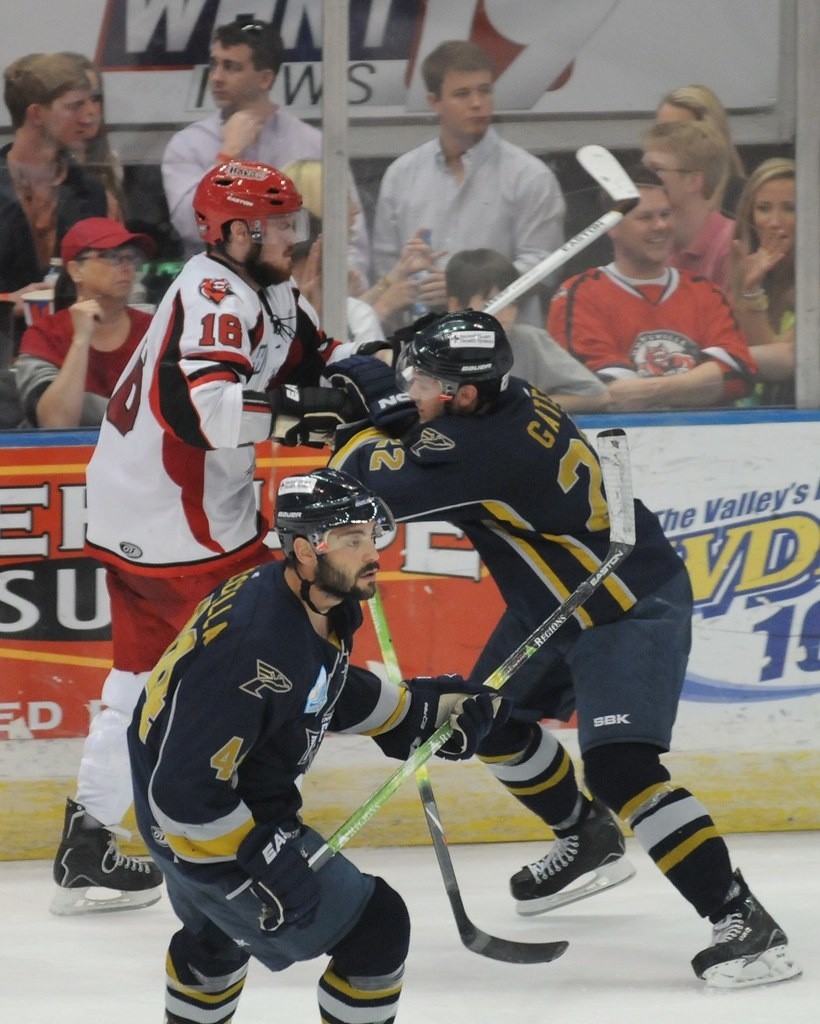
[690,867,802,989]
[49,794,163,915]
[509,779,637,916]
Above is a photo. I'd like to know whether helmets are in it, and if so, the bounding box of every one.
[274,467,381,561]
[406,309,514,404]
[192,161,302,244]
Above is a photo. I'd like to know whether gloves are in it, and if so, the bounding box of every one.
[321,355,419,438]
[268,383,365,453]
[221,821,322,937]
[372,674,515,761]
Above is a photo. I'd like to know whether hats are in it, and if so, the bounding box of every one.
[61,217,158,266]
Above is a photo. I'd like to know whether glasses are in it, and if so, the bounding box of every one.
[648,165,694,175]
[241,24,265,45]
[73,249,142,269]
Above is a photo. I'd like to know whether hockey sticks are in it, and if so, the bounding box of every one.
[480,143,645,317]
[365,583,570,967]
[260,425,640,923]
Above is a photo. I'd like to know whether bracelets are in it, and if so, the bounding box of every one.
[742,294,769,311]
[379,276,391,288]
[216,153,234,164]
[743,289,766,300]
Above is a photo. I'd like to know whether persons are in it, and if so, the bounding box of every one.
[279,157,448,344]
[640,118,798,407]
[446,248,613,414]
[544,165,759,415]
[371,40,565,329]
[324,311,803,987]
[66,52,125,225]
[127,468,514,1024]
[51,161,474,915]
[0,52,108,356]
[15,218,153,430]
[658,84,750,220]
[160,21,370,297]
